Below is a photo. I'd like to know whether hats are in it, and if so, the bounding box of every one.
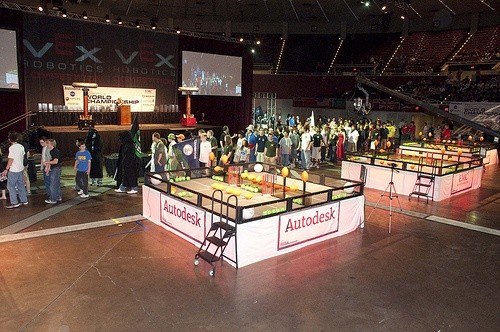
[167,133,175,140]
[176,134,185,139]
[245,124,254,132]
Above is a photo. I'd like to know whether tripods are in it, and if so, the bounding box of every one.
[364,165,404,233]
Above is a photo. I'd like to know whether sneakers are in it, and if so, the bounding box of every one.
[127,189,137,193]
[114,188,126,193]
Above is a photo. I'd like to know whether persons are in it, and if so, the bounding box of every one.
[145,112,499,178]
[43,139,63,203]
[74,142,92,197]
[40,136,51,195]
[3,133,28,209]
[114,132,138,194]
[0,143,8,200]
[71,139,85,192]
[17,133,32,194]
[85,129,104,187]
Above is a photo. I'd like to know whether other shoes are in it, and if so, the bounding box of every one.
[79,194,90,198]
[6,201,30,209]
[77,189,84,195]
[44,196,63,204]
[90,183,102,187]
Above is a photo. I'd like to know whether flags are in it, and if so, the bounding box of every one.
[131,114,142,159]
